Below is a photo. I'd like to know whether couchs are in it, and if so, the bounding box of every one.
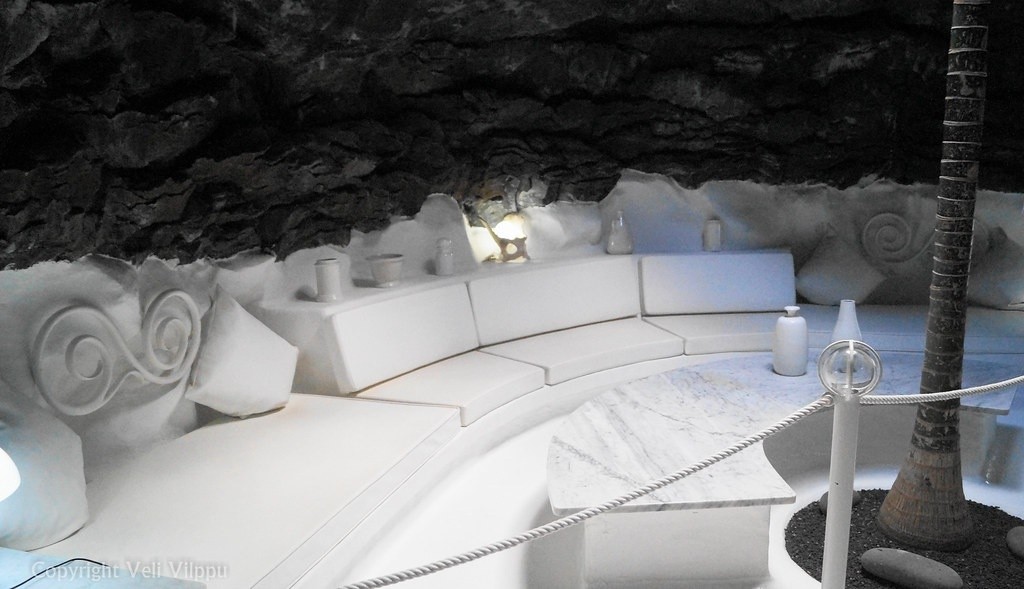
[272,248,1024,401]
[0,256,461,589]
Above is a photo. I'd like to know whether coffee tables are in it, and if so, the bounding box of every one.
[543,356,1024,581]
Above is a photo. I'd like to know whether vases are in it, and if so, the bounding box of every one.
[704,219,722,252]
[607,211,635,254]
[314,258,344,301]
[833,299,863,372]
[772,305,810,376]
[367,254,404,288]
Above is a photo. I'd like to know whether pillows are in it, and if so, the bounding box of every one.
[960,226,1024,312]
[183,282,300,421]
[794,230,886,306]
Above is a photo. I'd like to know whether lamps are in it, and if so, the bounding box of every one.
[477,212,531,261]
